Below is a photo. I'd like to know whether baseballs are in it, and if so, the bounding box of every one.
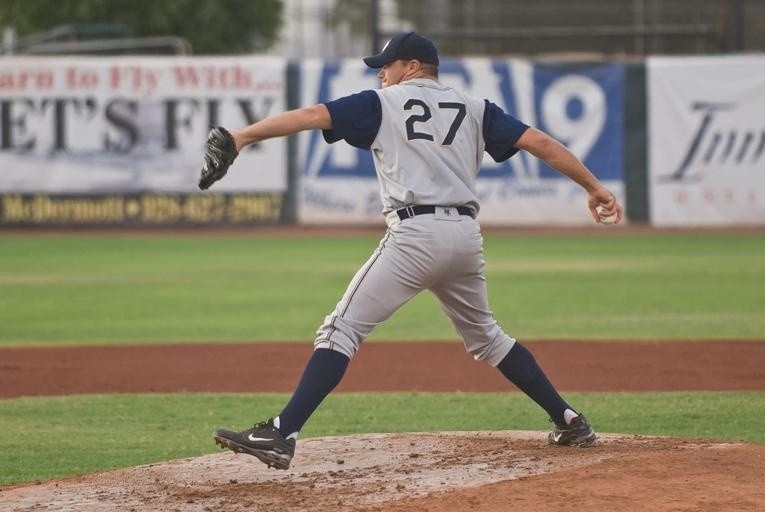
[599,208,617,224]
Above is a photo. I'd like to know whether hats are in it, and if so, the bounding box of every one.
[362,31,440,68]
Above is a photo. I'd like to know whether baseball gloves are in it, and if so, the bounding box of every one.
[199,126,238,190]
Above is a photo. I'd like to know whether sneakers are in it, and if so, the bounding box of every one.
[548,412,597,448]
[212,418,296,470]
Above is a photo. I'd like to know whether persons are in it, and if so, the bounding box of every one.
[199,32,623,471]
